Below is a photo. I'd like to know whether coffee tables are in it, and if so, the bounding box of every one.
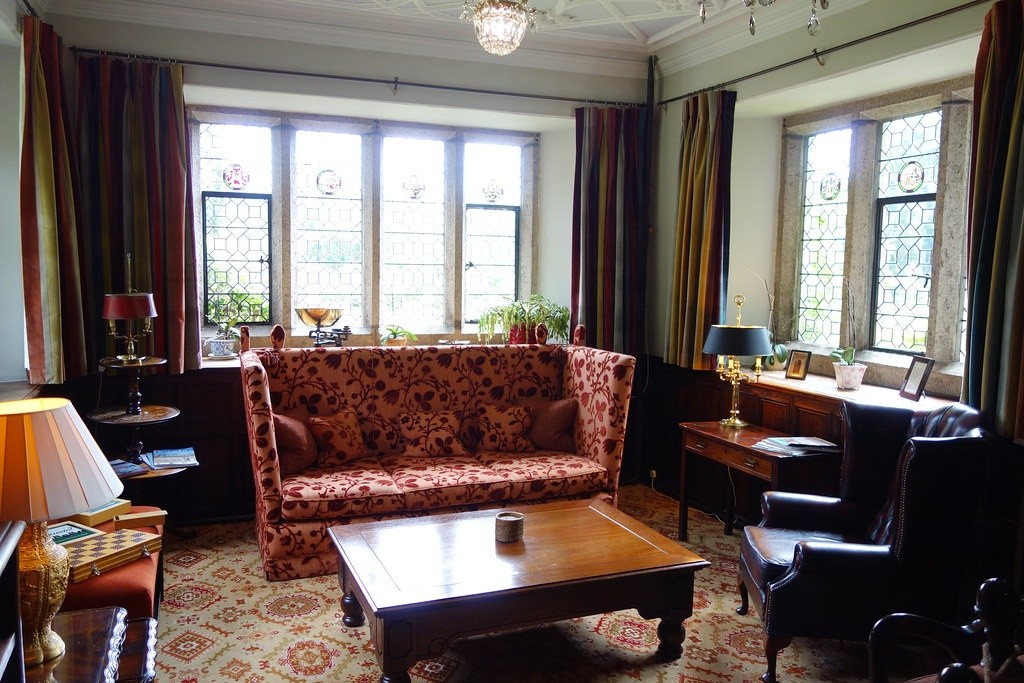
[326,496,711,682]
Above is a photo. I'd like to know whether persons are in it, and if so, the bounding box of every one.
[793,362,798,373]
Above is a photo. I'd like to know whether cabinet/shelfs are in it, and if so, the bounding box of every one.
[692,364,954,442]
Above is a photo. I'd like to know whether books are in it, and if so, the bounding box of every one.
[109,447,200,478]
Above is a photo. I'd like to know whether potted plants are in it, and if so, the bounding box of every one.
[207,308,241,355]
[475,294,567,346]
[830,348,870,391]
[379,324,418,347]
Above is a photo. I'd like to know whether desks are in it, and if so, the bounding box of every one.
[41,502,168,617]
[675,418,840,542]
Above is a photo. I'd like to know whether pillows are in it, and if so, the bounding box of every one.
[308,407,370,465]
[268,412,318,476]
[471,402,536,455]
[527,396,579,455]
[392,407,467,459]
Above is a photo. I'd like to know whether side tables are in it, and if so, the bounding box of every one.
[87,356,193,482]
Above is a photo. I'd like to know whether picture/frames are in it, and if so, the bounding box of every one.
[786,349,810,380]
[46,518,105,546]
[900,355,934,401]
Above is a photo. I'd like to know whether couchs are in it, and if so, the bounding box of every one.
[239,345,633,580]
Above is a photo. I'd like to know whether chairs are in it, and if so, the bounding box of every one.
[736,401,1002,683]
[863,572,1024,683]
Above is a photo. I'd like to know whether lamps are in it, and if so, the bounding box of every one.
[293,307,350,347]
[103,294,158,360]
[1,397,126,663]
[454,0,547,55]
[701,323,774,428]
